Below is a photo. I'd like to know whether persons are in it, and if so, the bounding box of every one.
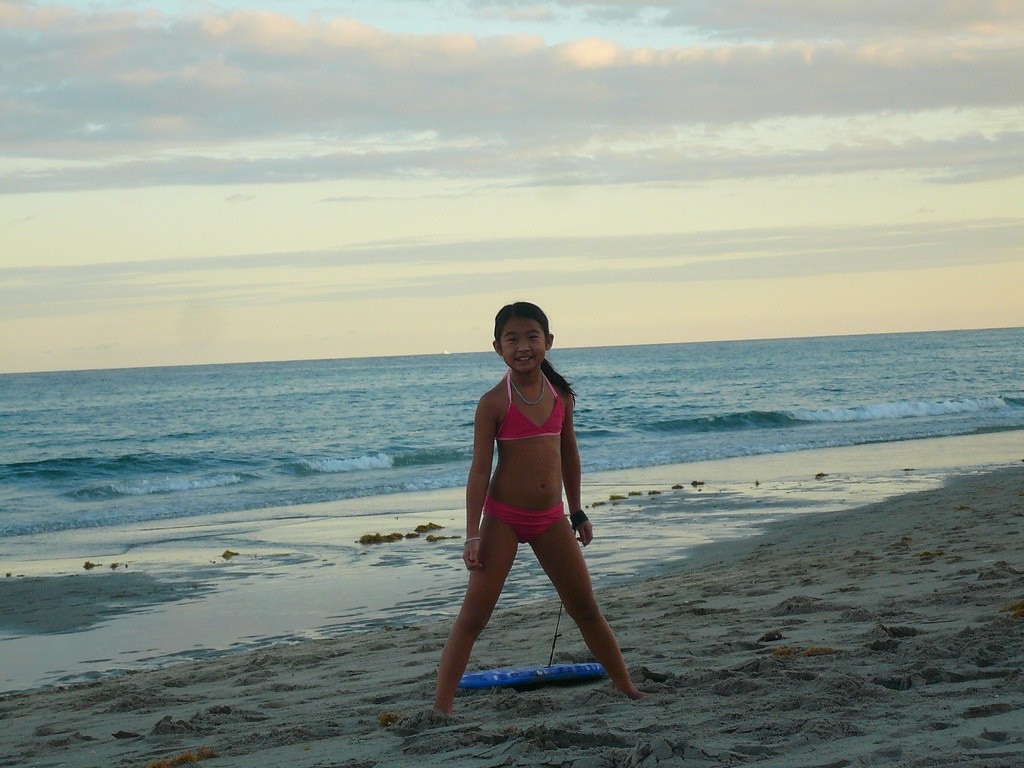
[431,302,651,717]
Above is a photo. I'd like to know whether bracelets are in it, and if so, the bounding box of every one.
[569,510,589,527]
[462,536,481,548]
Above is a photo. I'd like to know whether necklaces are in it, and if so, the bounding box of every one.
[510,370,545,405]
